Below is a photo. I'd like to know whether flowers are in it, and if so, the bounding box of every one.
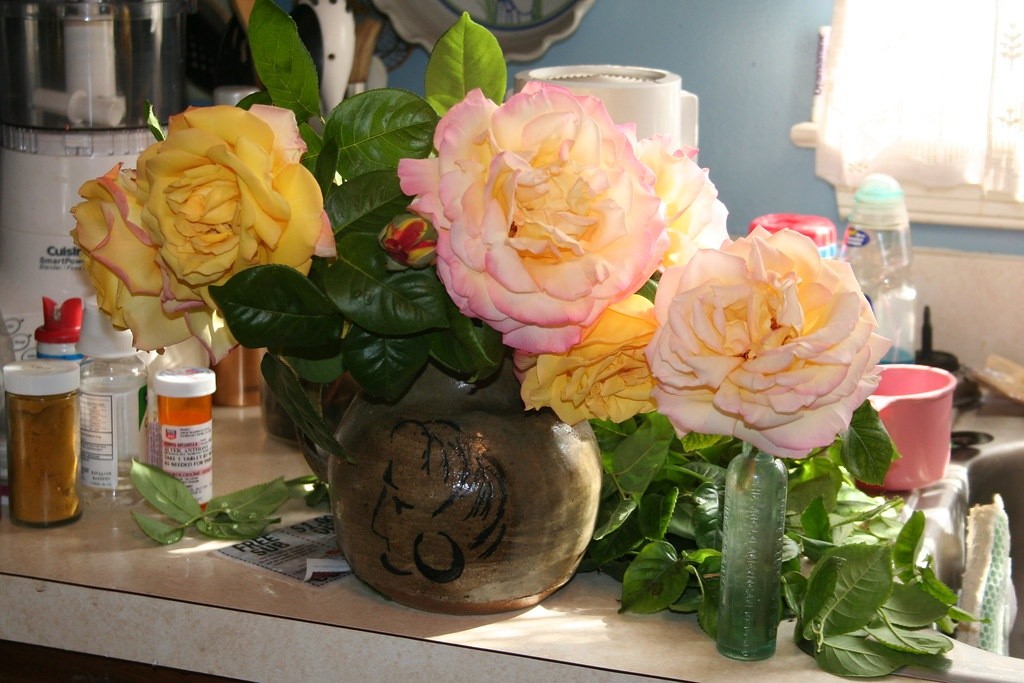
[71,0,973,680]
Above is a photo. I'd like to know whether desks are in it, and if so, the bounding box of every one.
[0,399,1024,683]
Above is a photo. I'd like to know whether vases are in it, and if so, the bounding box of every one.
[332,373,603,614]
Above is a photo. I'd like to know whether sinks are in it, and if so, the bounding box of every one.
[888,440,1023,683]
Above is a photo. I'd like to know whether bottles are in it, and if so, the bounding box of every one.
[748,213,838,263]
[3,359,84,527]
[35,295,92,365]
[75,356,152,512]
[153,365,217,512]
[841,172,918,366]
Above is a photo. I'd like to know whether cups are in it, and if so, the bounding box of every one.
[855,362,957,492]
[77,295,144,358]
